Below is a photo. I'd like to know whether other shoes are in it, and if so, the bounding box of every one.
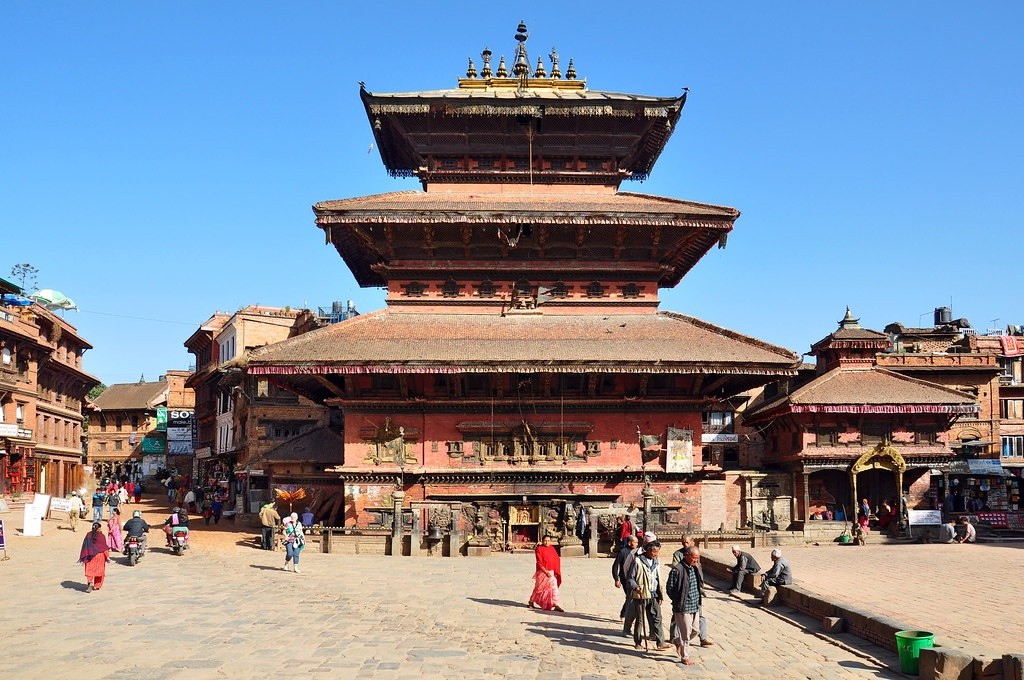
[700,640,714,645]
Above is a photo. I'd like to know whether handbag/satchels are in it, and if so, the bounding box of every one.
[297,537,306,547]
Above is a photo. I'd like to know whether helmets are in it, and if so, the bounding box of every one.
[133,510,141,517]
[173,507,180,513]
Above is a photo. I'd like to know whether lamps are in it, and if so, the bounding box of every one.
[234,385,252,404]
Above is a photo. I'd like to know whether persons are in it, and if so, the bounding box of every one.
[80,522,111,592]
[300,507,314,526]
[107,508,124,551]
[614,515,633,548]
[282,512,305,573]
[612,532,714,664]
[528,535,564,612]
[123,510,149,554]
[960,517,976,543]
[754,549,793,598]
[876,499,897,529]
[938,520,957,543]
[165,474,204,508]
[727,545,761,593]
[855,499,871,546]
[68,491,81,532]
[92,479,142,523]
[201,494,222,526]
[165,506,189,547]
[260,497,280,549]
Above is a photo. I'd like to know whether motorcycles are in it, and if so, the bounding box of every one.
[123,524,151,566]
[163,518,190,556]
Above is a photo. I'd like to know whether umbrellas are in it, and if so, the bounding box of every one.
[31,289,78,318]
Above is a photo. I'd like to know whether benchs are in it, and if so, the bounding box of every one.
[700,551,765,591]
[778,581,1003,680]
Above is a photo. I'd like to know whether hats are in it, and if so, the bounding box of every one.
[643,531,657,542]
[771,549,782,558]
[732,545,740,551]
[293,538,300,548]
[644,541,661,551]
